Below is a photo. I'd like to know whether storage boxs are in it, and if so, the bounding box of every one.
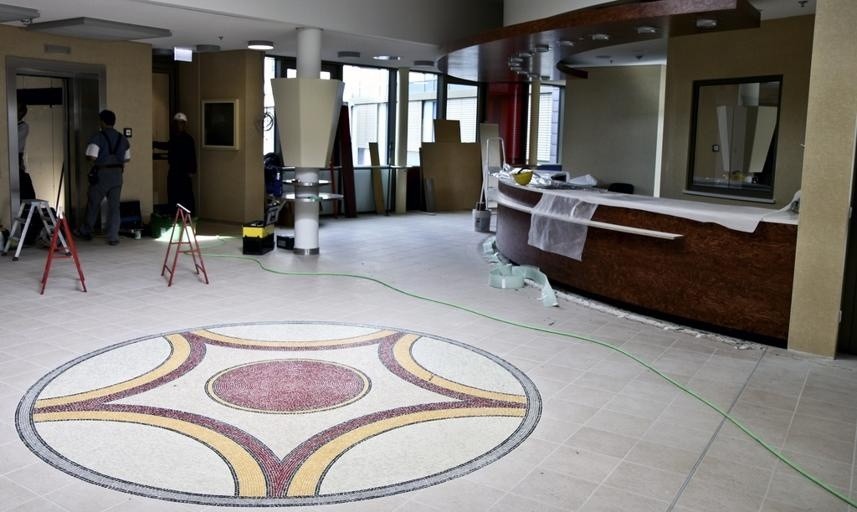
[241,220,274,255]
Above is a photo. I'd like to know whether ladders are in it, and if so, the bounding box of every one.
[40,211,88,296]
[479,136,507,215]
[3,198,72,261]
[161,203,209,288]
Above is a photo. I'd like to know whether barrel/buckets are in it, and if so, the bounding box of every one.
[472,209,491,233]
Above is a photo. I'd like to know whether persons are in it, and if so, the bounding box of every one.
[154,112,197,222]
[18,102,43,243]
[74,109,130,245]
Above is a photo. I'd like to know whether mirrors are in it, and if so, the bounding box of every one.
[681,74,782,205]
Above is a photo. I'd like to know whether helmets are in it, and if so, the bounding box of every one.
[173,112,188,123]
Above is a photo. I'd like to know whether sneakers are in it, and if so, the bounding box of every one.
[72,227,91,241]
[109,240,120,245]
